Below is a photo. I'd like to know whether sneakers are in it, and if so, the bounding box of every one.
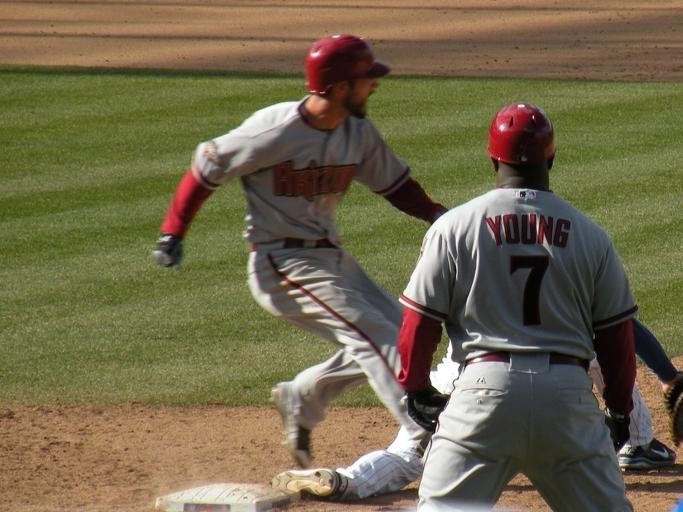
[271,467,349,496]
[617,435,677,470]
[271,388,314,468]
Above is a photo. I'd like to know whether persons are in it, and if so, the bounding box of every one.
[396,100,640,510]
[266,317,683,499]
[150,33,452,462]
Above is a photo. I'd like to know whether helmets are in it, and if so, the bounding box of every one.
[486,102,557,163]
[305,33,391,95]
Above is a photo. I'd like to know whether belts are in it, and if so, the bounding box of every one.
[464,349,591,371]
[249,239,336,251]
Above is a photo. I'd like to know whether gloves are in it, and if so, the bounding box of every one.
[152,234,184,266]
[603,412,630,451]
